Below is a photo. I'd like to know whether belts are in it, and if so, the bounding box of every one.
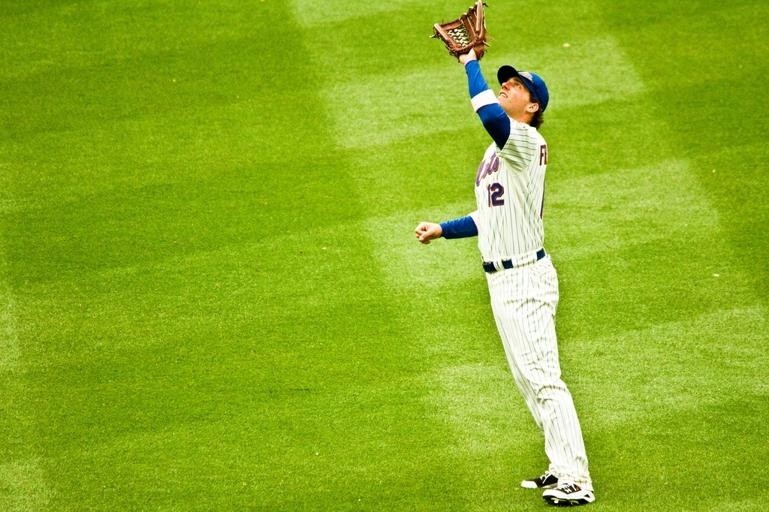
[482,247,546,274]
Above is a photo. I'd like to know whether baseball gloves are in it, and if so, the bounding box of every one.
[429,0,492,62]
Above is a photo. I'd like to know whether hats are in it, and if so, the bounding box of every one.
[497,65,549,112]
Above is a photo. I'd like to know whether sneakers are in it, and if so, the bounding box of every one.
[543,481,596,506]
[520,473,559,489]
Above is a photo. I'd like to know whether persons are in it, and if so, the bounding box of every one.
[414,0,602,507]
